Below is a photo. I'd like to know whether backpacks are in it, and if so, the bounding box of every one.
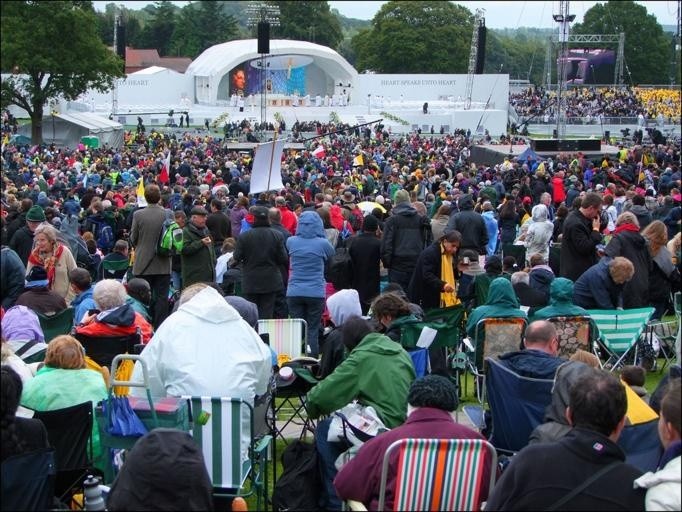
[84,215,115,249]
[155,208,184,257]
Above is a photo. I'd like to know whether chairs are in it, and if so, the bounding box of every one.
[342,438,497,511]
[482,357,555,455]
[648,291,682,374]
[171,395,273,511]
[103,260,129,280]
[233,279,242,296]
[546,315,593,362]
[253,319,309,446]
[462,318,528,403]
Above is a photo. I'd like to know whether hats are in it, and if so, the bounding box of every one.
[400,166,410,173]
[408,376,459,412]
[190,208,208,216]
[338,190,356,204]
[511,182,522,189]
[247,206,270,220]
[25,266,48,284]
[26,204,48,223]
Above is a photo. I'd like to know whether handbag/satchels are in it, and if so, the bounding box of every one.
[272,415,323,511]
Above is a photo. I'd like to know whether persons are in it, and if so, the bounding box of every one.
[284,210,337,359]
[9,225,35,270]
[529,359,589,444]
[485,320,570,456]
[82,240,102,284]
[231,68,247,94]
[332,374,500,511]
[425,194,435,218]
[391,123,462,188]
[105,427,213,512]
[220,269,241,295]
[624,194,652,231]
[104,205,119,241]
[329,205,353,233]
[206,199,231,258]
[26,224,77,307]
[305,315,417,512]
[551,205,569,243]
[233,206,288,319]
[2,304,50,364]
[176,193,196,218]
[365,89,409,111]
[0,108,43,198]
[0,198,11,228]
[215,237,236,284]
[651,195,675,221]
[0,214,25,312]
[578,129,682,188]
[640,219,682,321]
[128,282,272,464]
[381,188,434,292]
[502,255,520,281]
[569,197,583,211]
[275,195,297,236]
[98,240,133,282]
[604,183,616,195]
[314,192,332,211]
[248,194,258,205]
[317,288,362,379]
[190,117,267,199]
[315,204,330,229]
[457,248,479,308]
[604,211,653,308]
[0,364,50,463]
[124,196,137,210]
[80,196,111,254]
[83,231,105,259]
[172,210,186,289]
[601,194,618,235]
[268,118,338,195]
[230,196,249,238]
[338,119,391,190]
[443,193,489,257]
[44,207,56,224]
[538,191,555,221]
[340,191,364,232]
[228,88,261,111]
[462,124,551,193]
[406,230,464,309]
[181,206,217,288]
[511,271,548,317]
[14,265,67,317]
[371,292,424,343]
[530,276,599,341]
[475,255,511,305]
[349,214,381,316]
[465,276,530,366]
[430,205,452,240]
[572,254,635,310]
[578,190,588,199]
[509,83,601,125]
[268,207,293,319]
[103,127,190,192]
[551,142,578,197]
[483,369,648,512]
[18,334,108,472]
[68,267,100,326]
[620,365,651,407]
[633,377,682,512]
[292,203,303,220]
[63,192,81,216]
[130,182,176,333]
[373,283,424,333]
[644,189,657,210]
[663,205,682,240]
[255,193,271,208]
[613,186,631,216]
[602,84,681,126]
[159,195,173,210]
[498,199,521,244]
[624,184,638,199]
[43,142,102,196]
[518,196,532,227]
[125,278,152,324]
[408,191,428,217]
[667,232,682,265]
[7,198,33,245]
[105,190,118,206]
[523,203,555,268]
[436,89,468,114]
[72,277,154,373]
[529,253,554,275]
[290,90,350,110]
[480,201,498,256]
[37,191,50,206]
[6,202,21,228]
[671,188,682,207]
